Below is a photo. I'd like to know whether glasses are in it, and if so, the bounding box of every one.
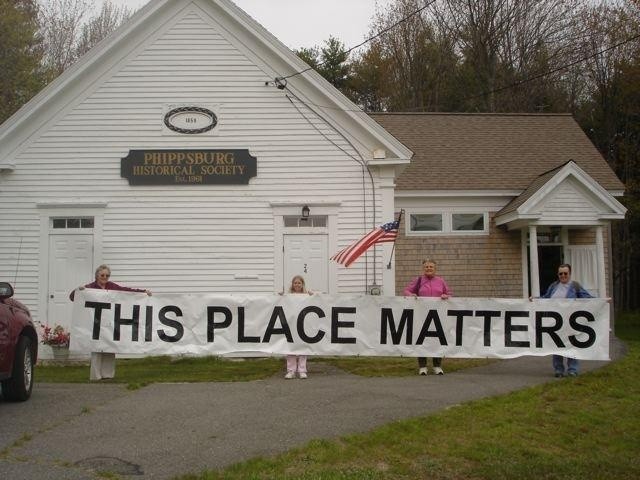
[558,271,569,276]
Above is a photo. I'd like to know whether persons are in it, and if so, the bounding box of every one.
[402,258,452,377]
[281,275,318,379]
[529,264,612,377]
[69,265,151,380]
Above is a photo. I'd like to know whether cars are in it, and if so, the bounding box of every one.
[0,278,40,406]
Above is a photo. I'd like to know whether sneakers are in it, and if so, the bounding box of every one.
[555,370,562,376]
[432,367,445,375]
[285,372,296,378]
[299,372,308,379]
[418,367,428,375]
[569,372,578,377]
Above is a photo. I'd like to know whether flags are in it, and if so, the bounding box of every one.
[330,221,400,268]
